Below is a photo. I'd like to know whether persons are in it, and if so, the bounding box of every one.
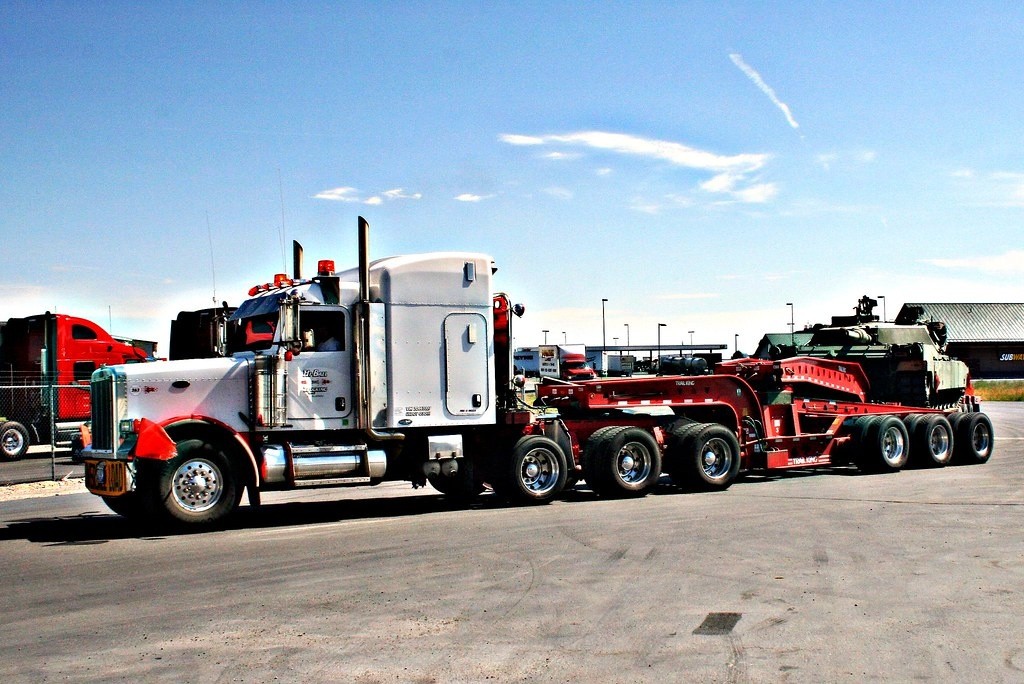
[313,320,341,351]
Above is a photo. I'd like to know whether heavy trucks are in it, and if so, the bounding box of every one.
[748,321,982,414]
[66,212,994,534]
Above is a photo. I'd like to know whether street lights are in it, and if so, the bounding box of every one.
[734,333,739,352]
[624,324,630,356]
[785,302,795,345]
[657,323,668,375]
[562,332,567,344]
[688,331,695,356]
[877,295,887,322]
[601,298,608,378]
[542,330,548,345]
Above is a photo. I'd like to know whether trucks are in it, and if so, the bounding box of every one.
[0,313,148,463]
[514,351,541,378]
[539,344,595,382]
